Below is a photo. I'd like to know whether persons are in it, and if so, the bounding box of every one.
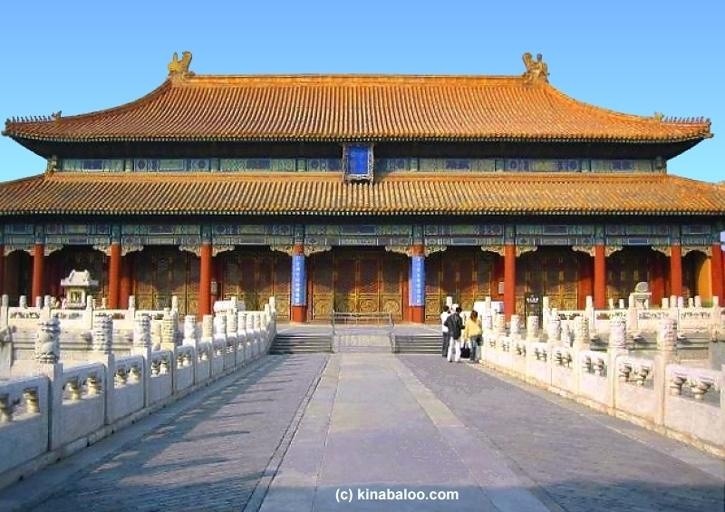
[50,293,68,318]
[441,305,482,364]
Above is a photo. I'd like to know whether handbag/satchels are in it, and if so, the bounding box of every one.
[461,347,470,358]
